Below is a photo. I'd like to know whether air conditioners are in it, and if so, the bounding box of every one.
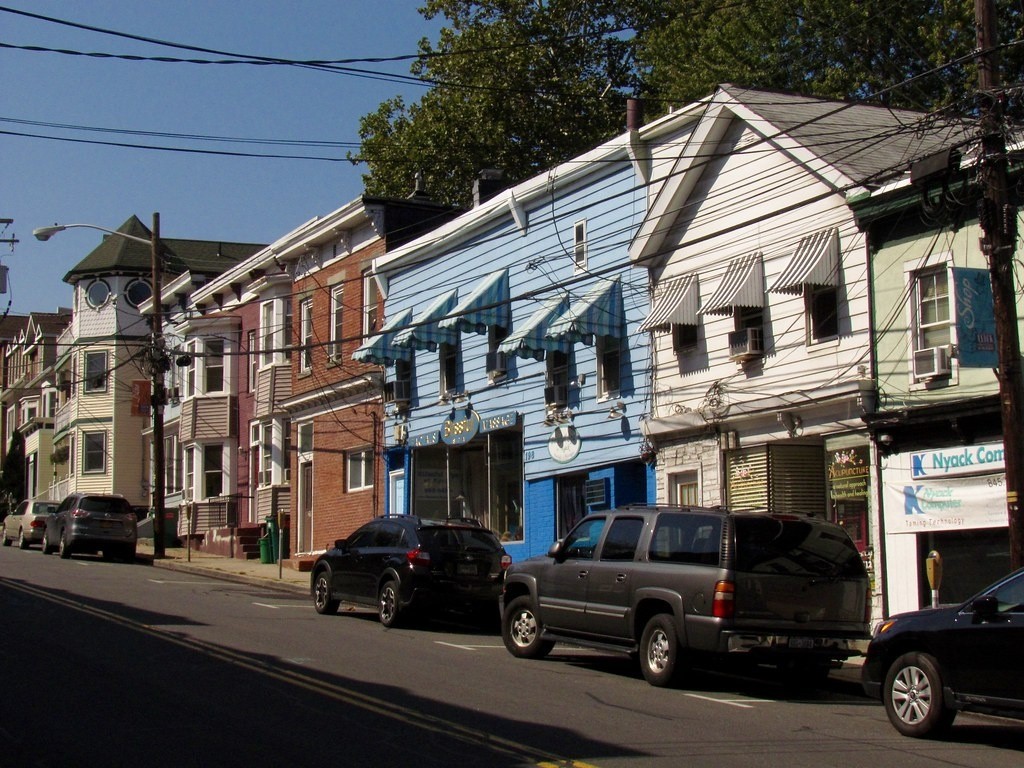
[383,380,410,403]
[393,425,407,441]
[727,328,765,363]
[912,344,953,379]
[543,385,567,408]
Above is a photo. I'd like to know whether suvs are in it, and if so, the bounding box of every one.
[497,502,873,700]
[309,513,513,635]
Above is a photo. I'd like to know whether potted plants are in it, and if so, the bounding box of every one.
[490,367,507,383]
[857,366,875,392]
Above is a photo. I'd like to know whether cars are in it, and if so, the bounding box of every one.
[859,565,1024,739]
[41,491,138,564]
[2,499,63,549]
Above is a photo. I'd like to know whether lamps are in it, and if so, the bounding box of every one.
[607,403,628,419]
[437,393,468,407]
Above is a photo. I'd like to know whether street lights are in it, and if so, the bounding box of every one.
[33,212,166,559]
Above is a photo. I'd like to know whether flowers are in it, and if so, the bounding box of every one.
[735,463,759,480]
[638,443,653,461]
[830,447,864,468]
